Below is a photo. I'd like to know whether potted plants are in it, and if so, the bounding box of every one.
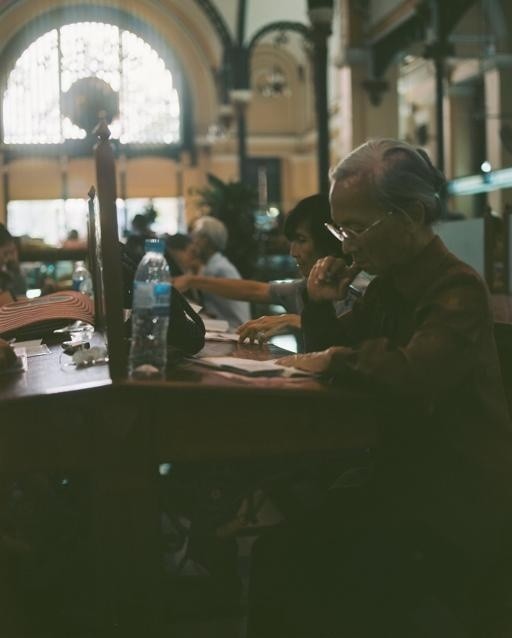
[194,171,261,280]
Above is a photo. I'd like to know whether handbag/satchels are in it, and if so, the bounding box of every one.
[121,283,207,362]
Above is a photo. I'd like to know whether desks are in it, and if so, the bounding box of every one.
[1,311,329,473]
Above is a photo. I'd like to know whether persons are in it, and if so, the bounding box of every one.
[63,229,88,250]
[119,204,251,334]
[1,222,56,296]
[168,191,346,356]
[246,138,512,638]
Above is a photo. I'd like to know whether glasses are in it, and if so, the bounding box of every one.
[322,207,401,243]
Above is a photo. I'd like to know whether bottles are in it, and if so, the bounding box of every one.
[128,232,175,386]
[70,259,93,301]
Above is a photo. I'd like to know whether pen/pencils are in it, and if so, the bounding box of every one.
[326,277,361,298]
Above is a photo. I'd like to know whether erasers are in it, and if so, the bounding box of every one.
[61,340,90,353]
